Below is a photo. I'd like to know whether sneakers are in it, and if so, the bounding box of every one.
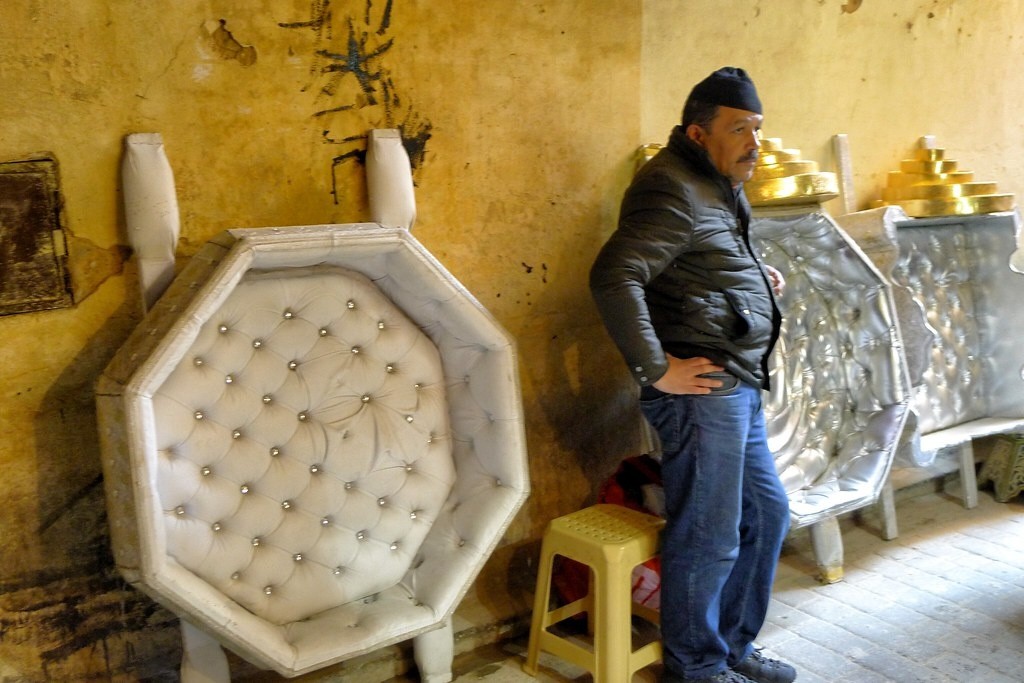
[662,664,758,683]
[731,647,797,683]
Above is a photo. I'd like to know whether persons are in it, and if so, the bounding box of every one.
[589,66,800,683]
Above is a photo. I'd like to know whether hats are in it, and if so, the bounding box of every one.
[690,67,762,113]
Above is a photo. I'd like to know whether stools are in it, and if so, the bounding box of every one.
[523,503,667,683]
